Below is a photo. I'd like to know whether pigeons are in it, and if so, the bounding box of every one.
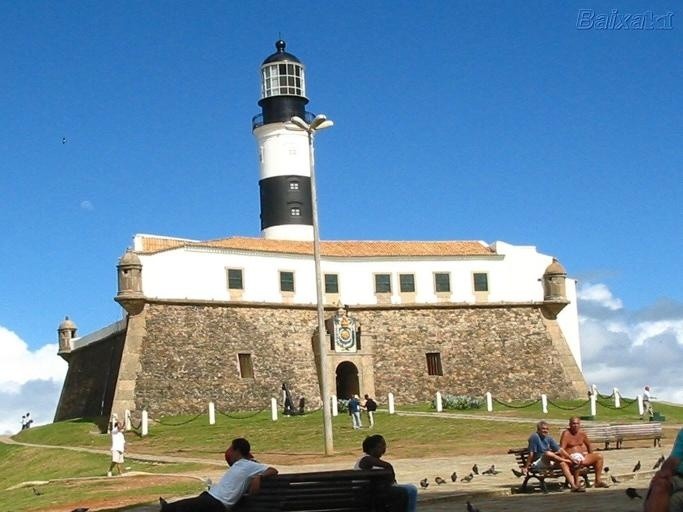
[466,500,480,512]
[511,469,525,478]
[653,458,661,469]
[420,464,497,490]
[625,487,642,500]
[611,476,621,486]
[604,466,609,473]
[633,460,641,472]
[659,454,665,465]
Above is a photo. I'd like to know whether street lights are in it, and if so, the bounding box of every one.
[284,113,335,456]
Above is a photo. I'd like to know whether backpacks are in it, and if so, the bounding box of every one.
[372,399,376,411]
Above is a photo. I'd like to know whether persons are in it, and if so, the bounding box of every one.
[553,416,610,492]
[105,415,128,478]
[156,437,278,512]
[347,393,361,429]
[349,432,417,512]
[20,416,25,430]
[642,427,682,511]
[603,438,623,450]
[24,412,31,428]
[639,385,656,420]
[652,437,661,447]
[521,421,583,493]
[360,393,374,428]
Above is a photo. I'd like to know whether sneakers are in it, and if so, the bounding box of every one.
[594,480,608,487]
[107,469,112,476]
[570,484,587,492]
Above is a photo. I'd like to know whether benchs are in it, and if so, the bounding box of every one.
[231,471,392,512]
[508,448,596,493]
[610,423,663,449]
[560,426,610,451]
